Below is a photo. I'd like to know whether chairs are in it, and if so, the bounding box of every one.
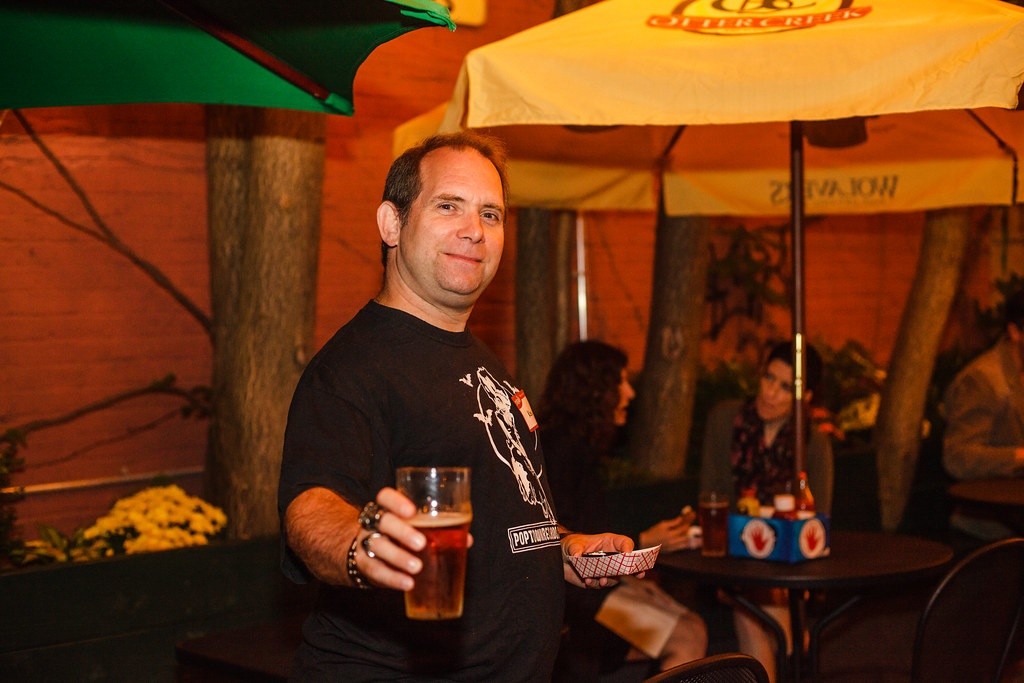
[910,536,1024,683]
[698,397,835,659]
[944,352,1024,545]
[642,652,770,682]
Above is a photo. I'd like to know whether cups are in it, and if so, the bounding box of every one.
[699,492,729,557]
[395,467,472,621]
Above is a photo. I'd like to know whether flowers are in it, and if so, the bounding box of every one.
[82,484,227,556]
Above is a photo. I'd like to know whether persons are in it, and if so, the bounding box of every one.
[927,288,1024,549]
[540,342,708,677]
[697,339,836,679]
[281,133,646,682]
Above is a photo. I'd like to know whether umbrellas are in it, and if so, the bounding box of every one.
[0,0,457,118]
[394,0,1024,509]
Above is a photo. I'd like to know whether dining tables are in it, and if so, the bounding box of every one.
[655,531,955,683]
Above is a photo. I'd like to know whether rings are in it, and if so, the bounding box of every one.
[361,532,381,560]
[358,501,387,530]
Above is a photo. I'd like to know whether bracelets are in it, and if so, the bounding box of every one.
[347,533,368,588]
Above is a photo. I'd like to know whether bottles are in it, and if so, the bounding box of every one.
[738,488,762,516]
[795,472,816,518]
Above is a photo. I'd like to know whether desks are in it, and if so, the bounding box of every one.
[174,614,630,683]
[947,476,1024,528]
